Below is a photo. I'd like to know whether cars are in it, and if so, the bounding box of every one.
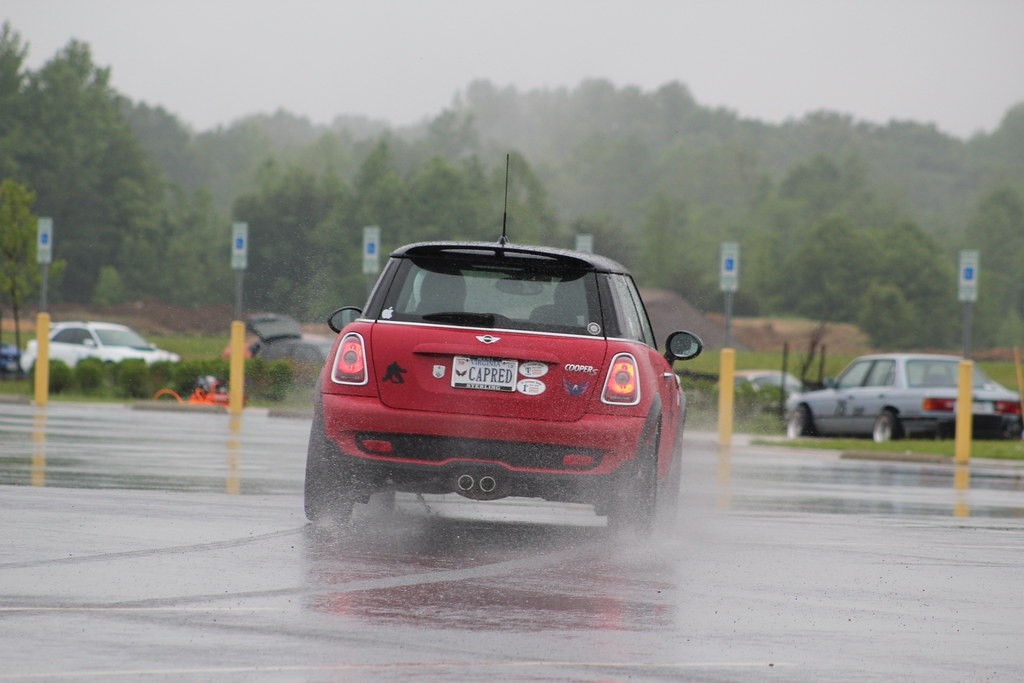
[246,312,333,365]
[0,341,25,380]
[20,319,183,377]
[712,368,812,402]
[786,352,1024,442]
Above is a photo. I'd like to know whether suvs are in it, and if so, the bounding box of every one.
[303,240,704,539]
